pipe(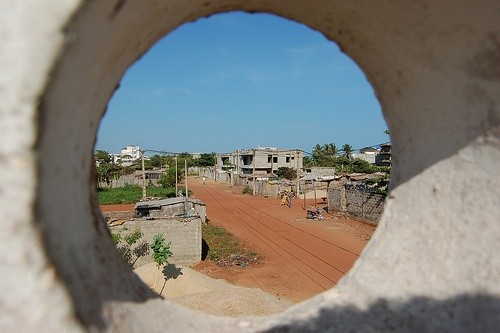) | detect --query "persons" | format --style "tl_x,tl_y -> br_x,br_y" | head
280,183 -> 291,208
